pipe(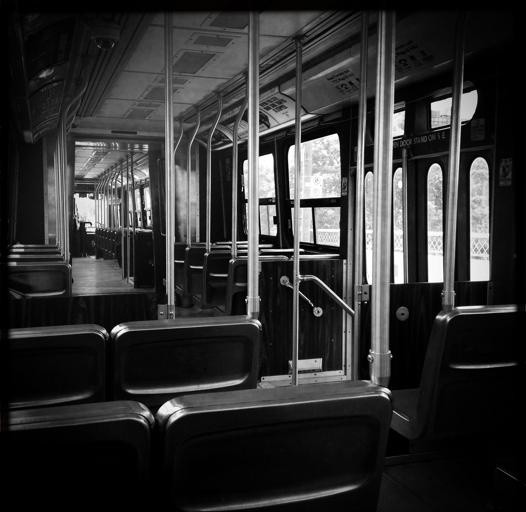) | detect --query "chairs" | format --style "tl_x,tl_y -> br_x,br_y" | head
2,324 -> 110,410
7,244 -> 73,323
108,315 -> 264,407
157,378 -> 396,512
168,239 -> 340,314
95,227 -> 150,271
1,401 -> 157,512
387,302 -> 521,444
